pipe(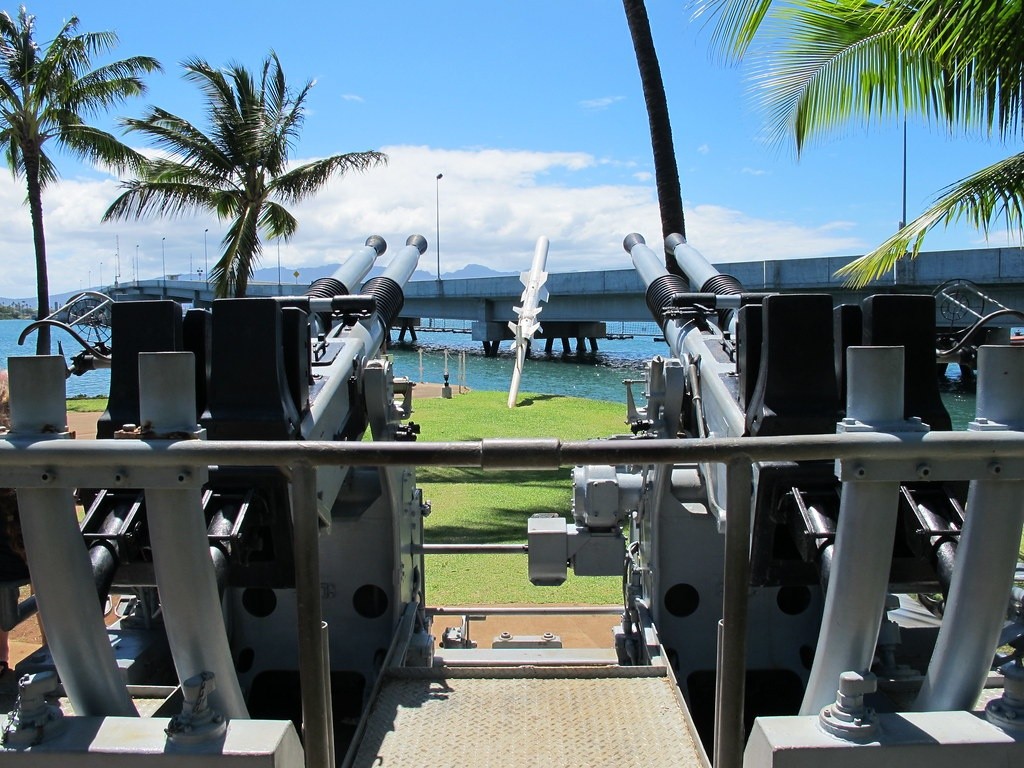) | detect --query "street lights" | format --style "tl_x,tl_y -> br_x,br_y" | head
437,174 -> 443,279
88,270 -> 92,292
163,237 -> 165,280
100,262 -> 103,286
204,228 -> 209,282
114,254 -> 117,275
138,244 -> 139,281
